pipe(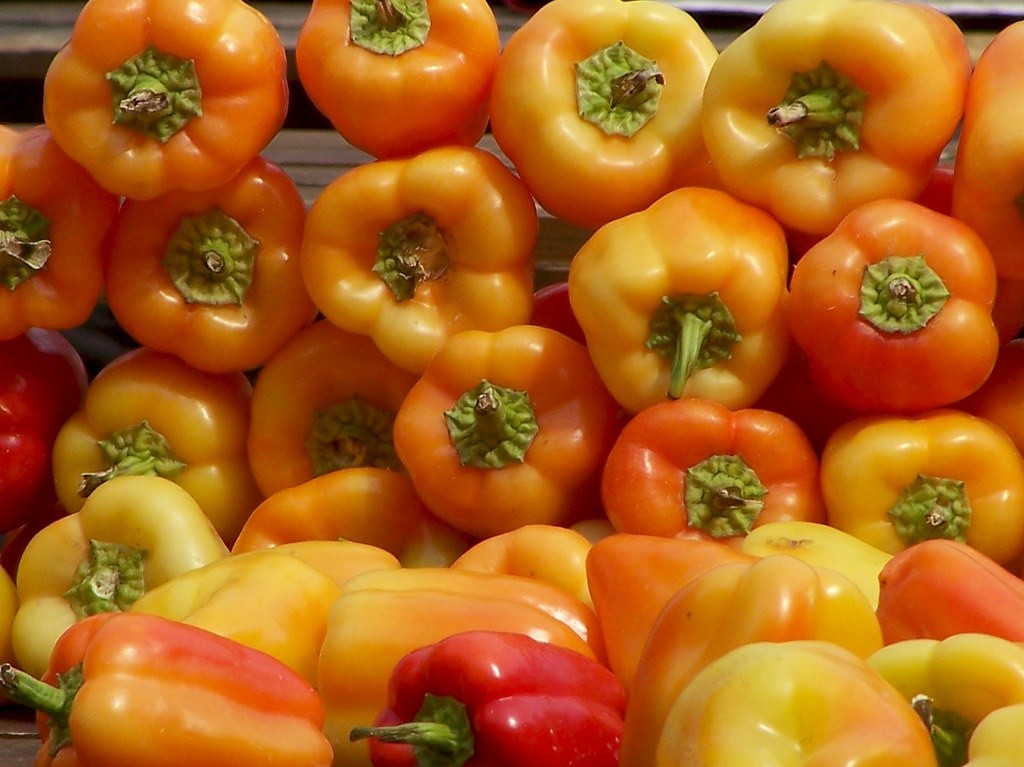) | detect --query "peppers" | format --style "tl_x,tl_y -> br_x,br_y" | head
0,0 -> 1024,767
